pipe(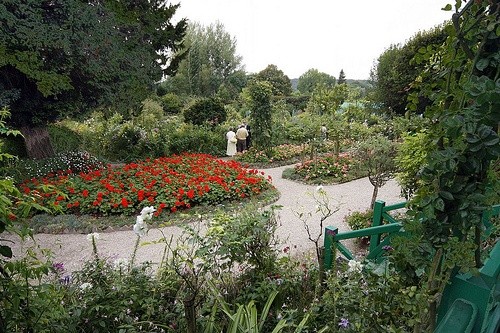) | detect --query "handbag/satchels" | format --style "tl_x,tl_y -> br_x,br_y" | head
230,136 -> 237,143
